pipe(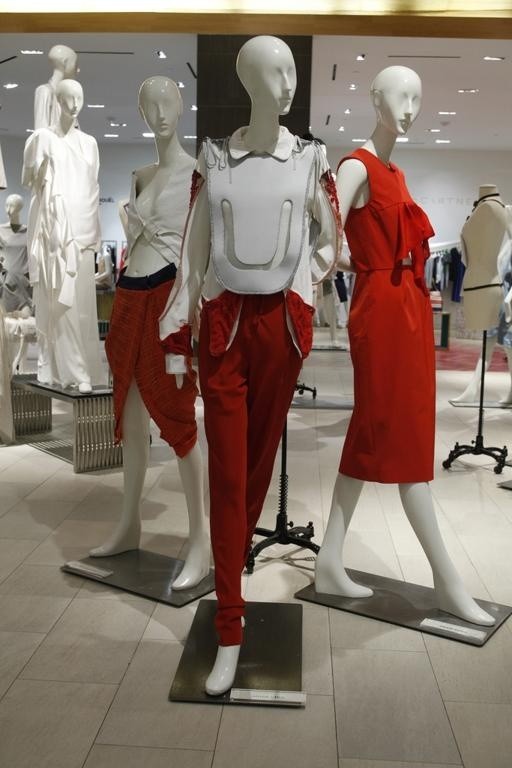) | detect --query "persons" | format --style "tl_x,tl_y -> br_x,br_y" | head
312,64 -> 498,630
89,74 -> 215,592
455,181 -> 506,336
32,41 -> 84,133
319,171 -> 350,349
0,192 -> 41,376
19,76 -> 114,392
447,204 -> 512,409
153,33 -> 344,697
